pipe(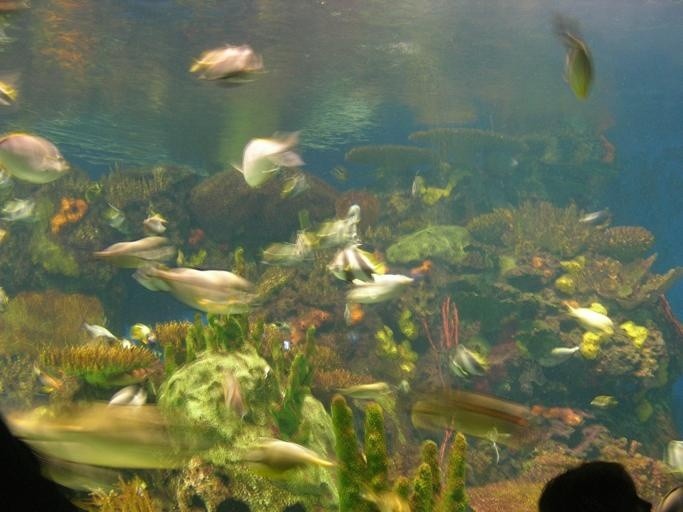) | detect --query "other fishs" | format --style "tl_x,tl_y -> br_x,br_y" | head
0,128 -> 614,504
